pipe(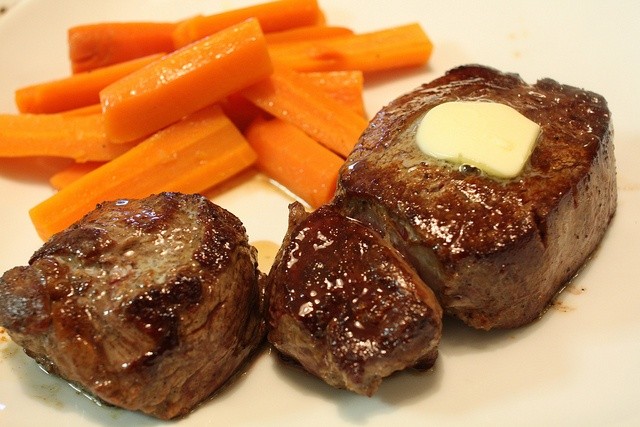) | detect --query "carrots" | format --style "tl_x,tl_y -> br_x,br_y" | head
0,0 -> 432,244
